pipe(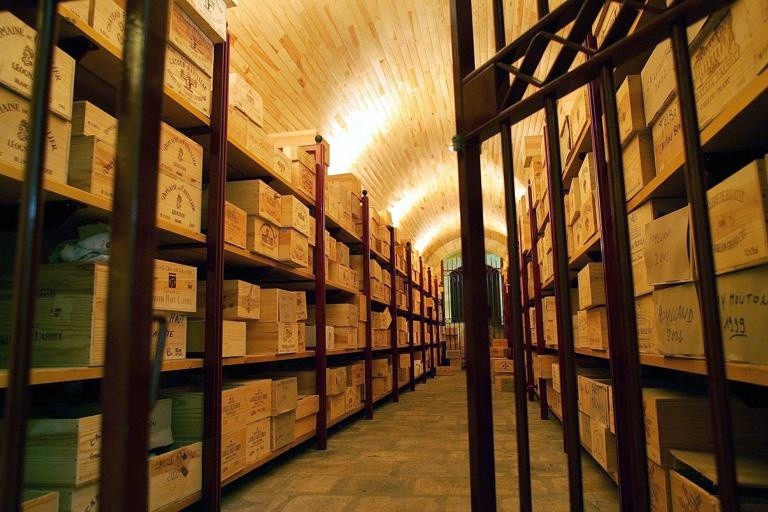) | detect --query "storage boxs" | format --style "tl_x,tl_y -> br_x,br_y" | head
367,197 -> 398,420
504,0 -> 768,512
322,145 -> 373,449
393,227 -> 444,405
1,1 -> 226,511
218,31 -> 325,510
